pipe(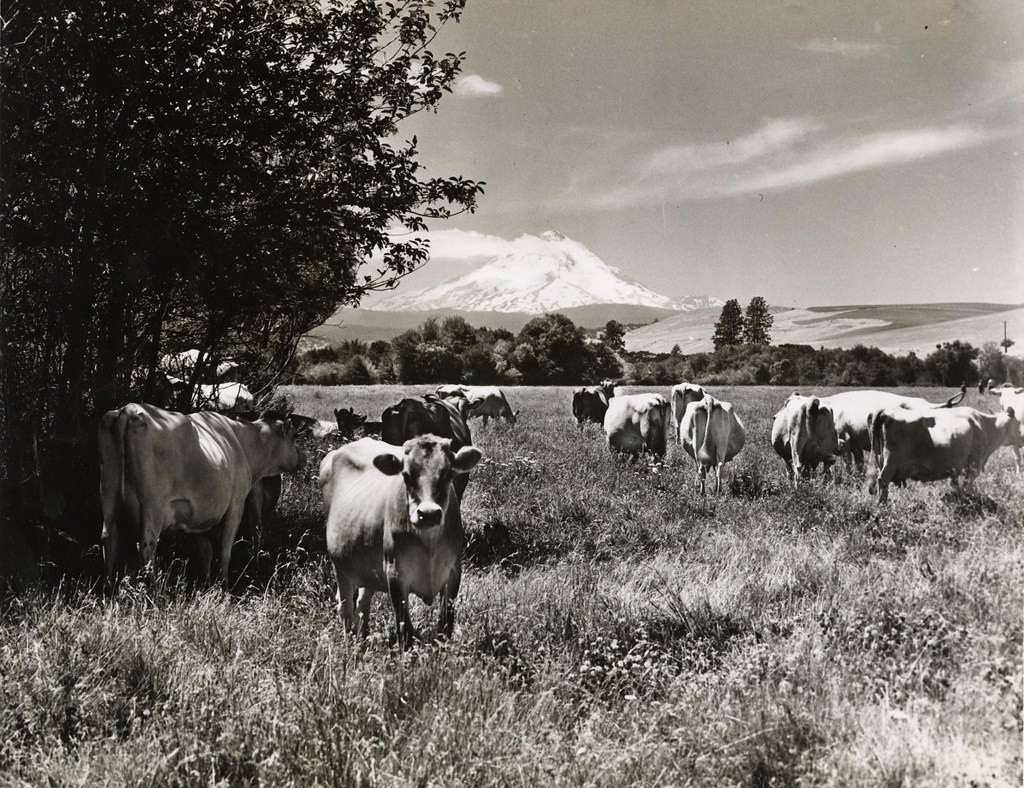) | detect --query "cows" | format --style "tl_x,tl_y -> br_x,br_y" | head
771,382 -> 1024,504
571,378 -> 746,494
98,348 -> 518,645
